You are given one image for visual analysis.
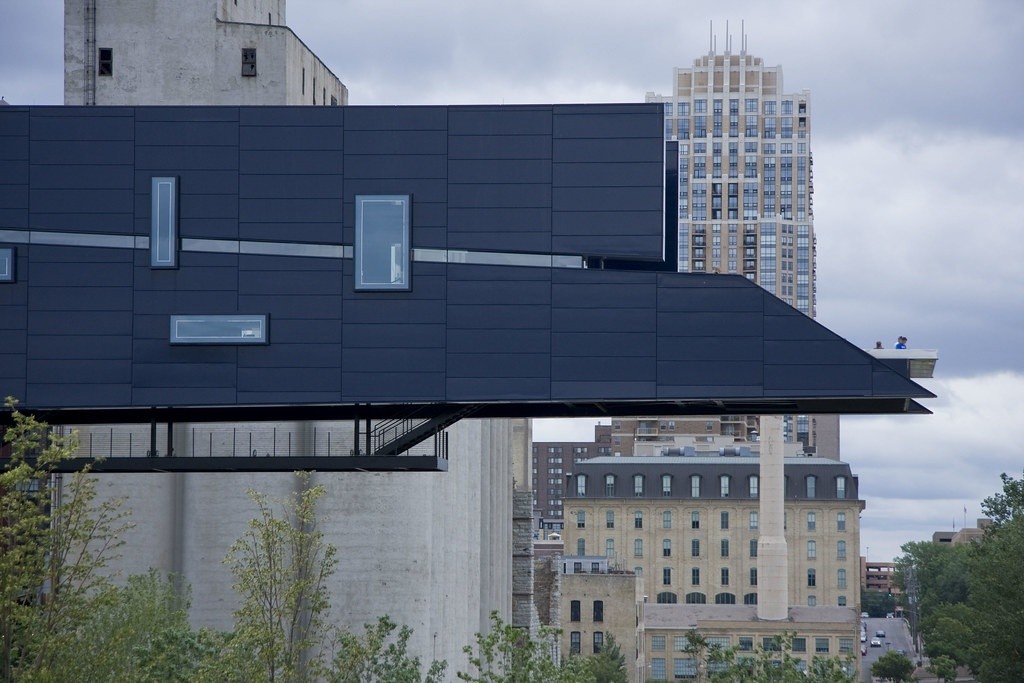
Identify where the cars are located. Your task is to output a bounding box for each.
[876,630,885,637]
[861,645,867,656]
[870,637,882,647]
[860,611,869,618]
[887,613,894,619]
[861,620,867,642]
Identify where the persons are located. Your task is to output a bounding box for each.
[895,335,908,349]
[874,341,883,349]
